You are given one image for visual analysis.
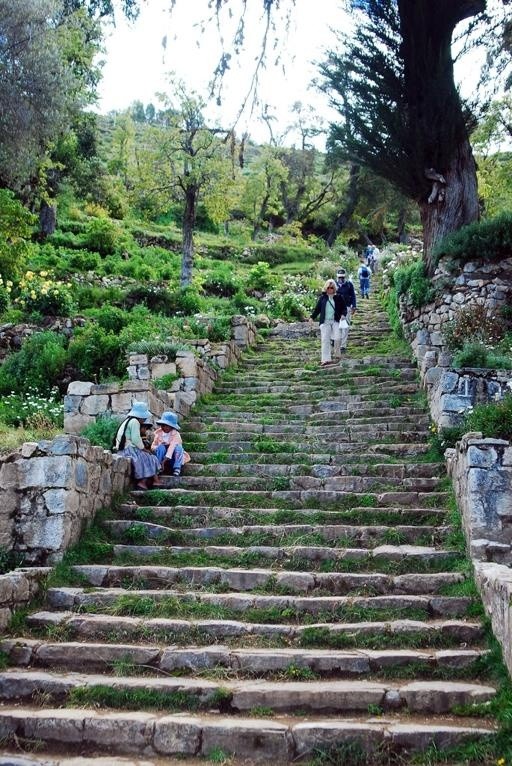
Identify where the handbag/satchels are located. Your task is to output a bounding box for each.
[339,319,349,329]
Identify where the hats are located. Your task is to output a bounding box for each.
[337,269,345,278]
[142,412,154,426]
[126,402,153,418]
[156,412,180,432]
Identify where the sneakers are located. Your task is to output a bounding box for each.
[173,469,180,475]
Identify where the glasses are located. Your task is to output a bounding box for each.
[327,287,333,289]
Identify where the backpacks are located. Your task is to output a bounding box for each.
[362,266,368,277]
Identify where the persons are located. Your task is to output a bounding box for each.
[140,412,154,449]
[116,402,166,489]
[330,269,356,350]
[358,243,380,300]
[309,279,347,366]
[151,412,191,476]
